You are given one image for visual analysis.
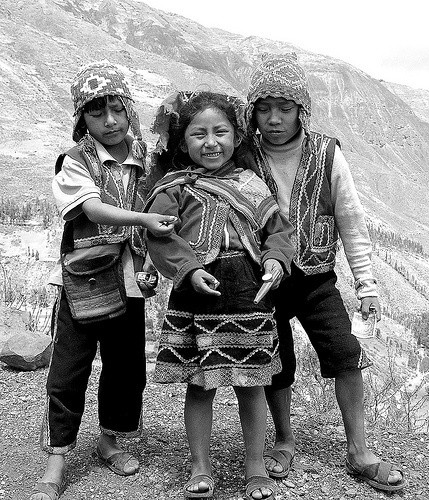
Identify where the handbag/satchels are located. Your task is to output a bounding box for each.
[62,252,127,324]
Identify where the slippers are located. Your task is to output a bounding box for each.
[27,481,67,500]
[262,447,296,477]
[344,458,407,490]
[96,444,139,476]
[243,467,277,500]
[183,469,215,498]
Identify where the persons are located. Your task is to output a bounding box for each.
[28,59,177,500]
[146,88,290,500]
[239,53,407,491]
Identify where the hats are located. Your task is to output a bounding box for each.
[70,62,148,160]
[245,51,312,154]
